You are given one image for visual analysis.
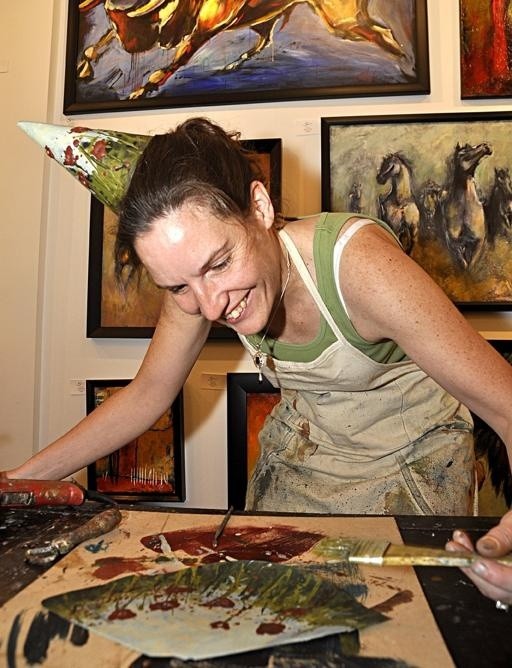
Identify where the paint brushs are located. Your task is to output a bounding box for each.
[214,505,234,539]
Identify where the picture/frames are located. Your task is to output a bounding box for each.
[227,372,281,511]
[86,138,282,339]
[320,110,512,312]
[63,0,430,116]
[85,379,186,502]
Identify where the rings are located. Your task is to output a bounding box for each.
[494,599,509,614]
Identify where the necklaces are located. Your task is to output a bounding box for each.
[244,253,292,380]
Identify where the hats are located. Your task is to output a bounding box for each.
[18,121,154,219]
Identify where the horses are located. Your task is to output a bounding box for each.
[416,178,442,241]
[484,167,511,237]
[439,142,493,276]
[375,152,421,259]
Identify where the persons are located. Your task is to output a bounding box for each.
[0,116,512,616]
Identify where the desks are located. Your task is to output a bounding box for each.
[0,501,512,668]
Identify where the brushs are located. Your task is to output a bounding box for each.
[314,537,512,567]
